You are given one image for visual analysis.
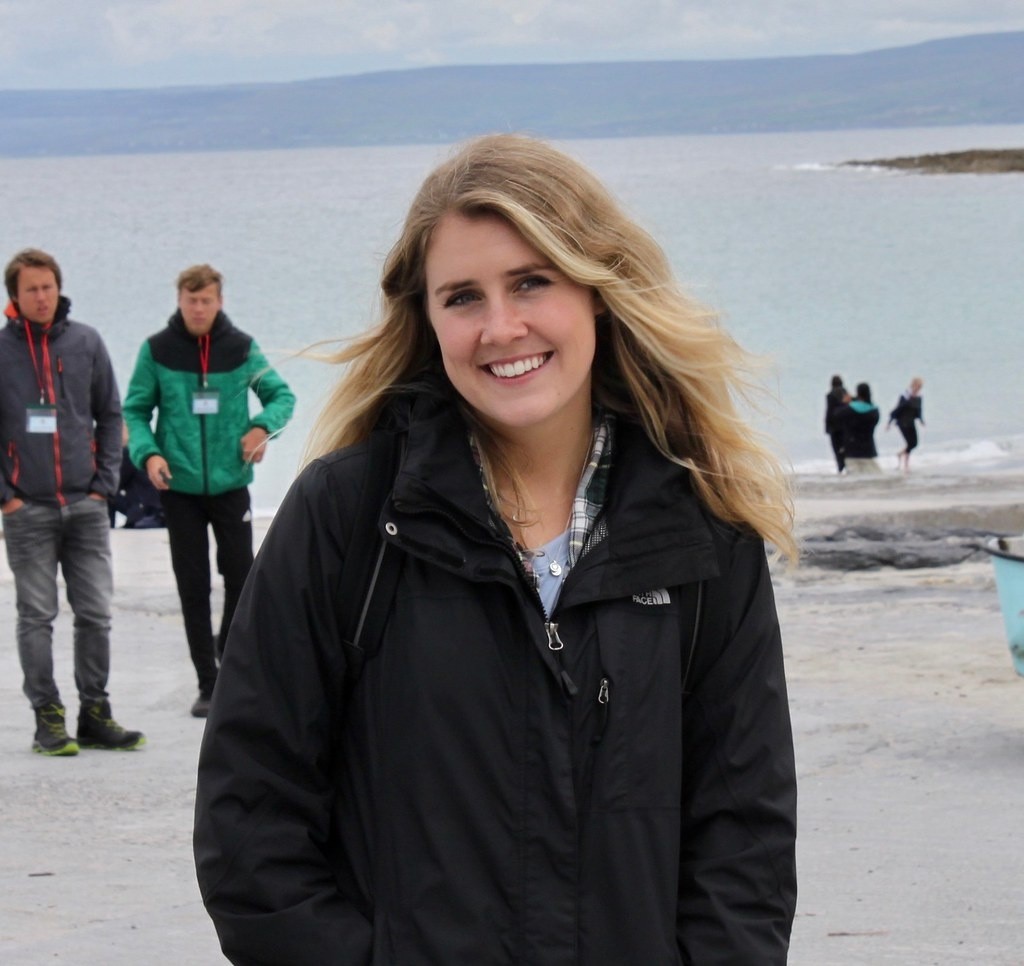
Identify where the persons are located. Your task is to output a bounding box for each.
[124,262,297,717]
[886,378,927,471]
[195,141,798,966]
[0,246,148,757]
[106,406,164,529]
[825,375,851,472]
[831,382,882,474]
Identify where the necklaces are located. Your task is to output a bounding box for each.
[480,420,591,575]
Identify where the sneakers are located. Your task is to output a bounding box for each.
[190,692,212,717]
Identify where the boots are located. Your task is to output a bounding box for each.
[30,704,80,758]
[75,700,146,749]
